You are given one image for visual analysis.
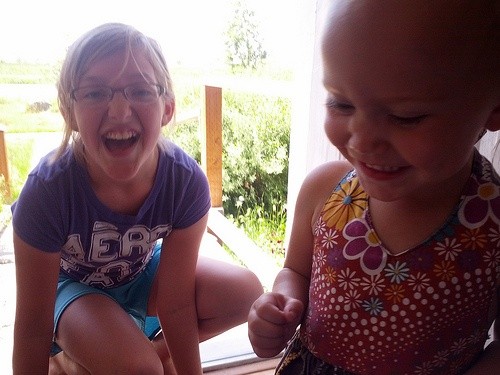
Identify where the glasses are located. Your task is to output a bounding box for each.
[67,82,165,108]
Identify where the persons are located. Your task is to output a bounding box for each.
[10,22,264,375]
[246,0,498,375]
